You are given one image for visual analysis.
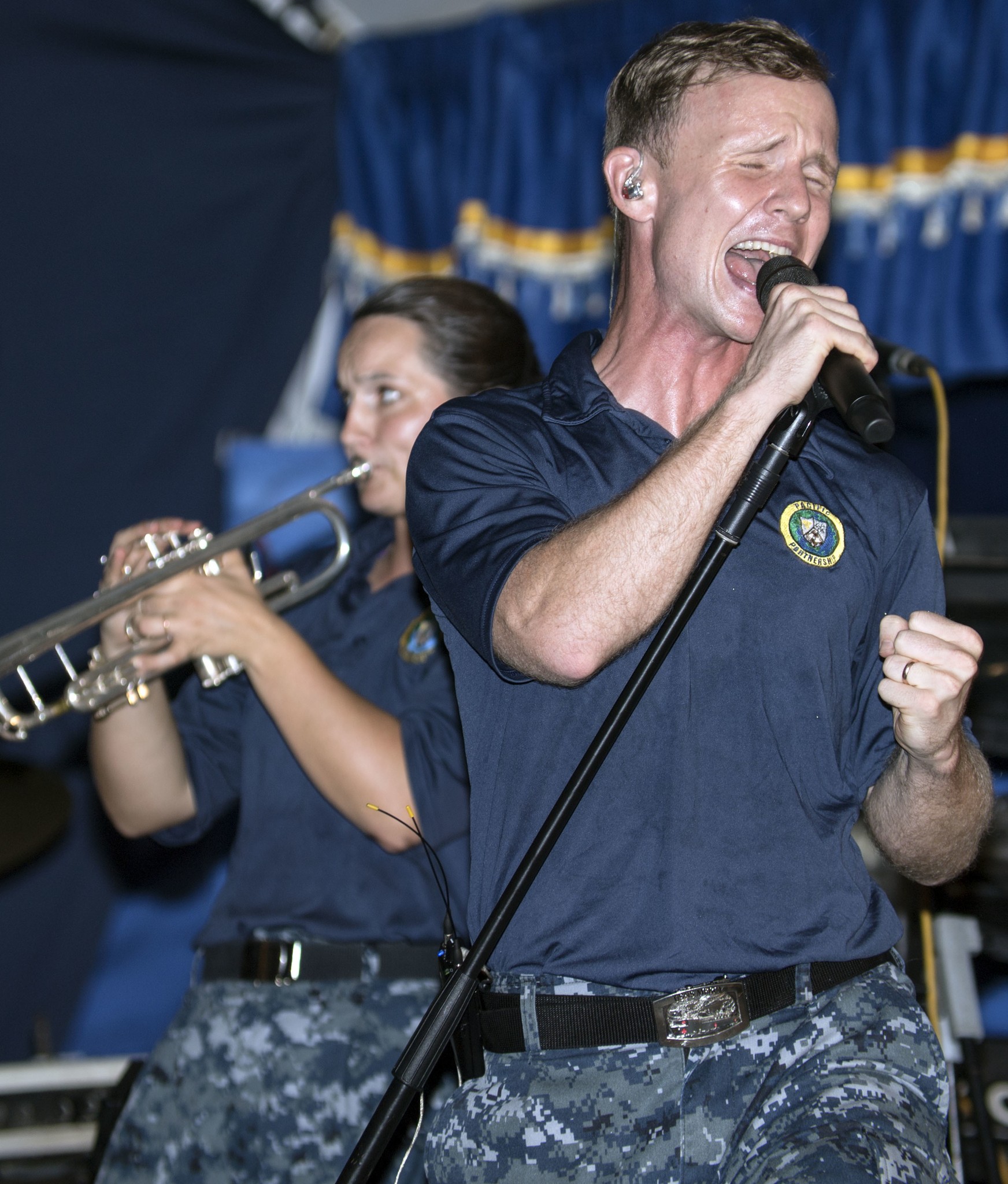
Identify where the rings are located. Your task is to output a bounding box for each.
[161,616,167,638]
[902,659,917,686]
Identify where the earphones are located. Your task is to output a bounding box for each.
[622,177,645,201]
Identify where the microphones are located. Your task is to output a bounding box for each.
[756,255,895,446]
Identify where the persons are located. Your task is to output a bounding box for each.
[407,15,997,1184]
[88,277,546,1184]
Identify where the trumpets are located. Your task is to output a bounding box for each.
[0,454,373,742]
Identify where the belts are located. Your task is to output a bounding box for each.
[203,936,438,984]
[475,947,891,1055]
